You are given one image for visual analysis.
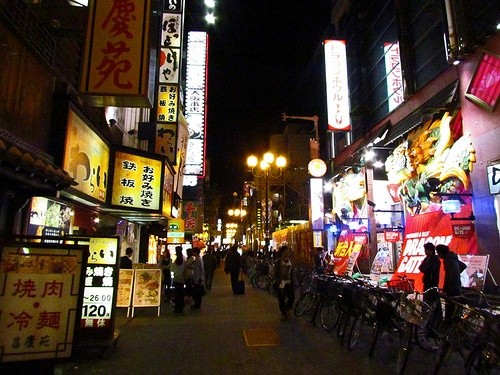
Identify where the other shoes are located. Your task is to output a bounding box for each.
[280,312,288,320]
[191,305,201,309]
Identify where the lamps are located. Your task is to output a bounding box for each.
[247,150,286,178]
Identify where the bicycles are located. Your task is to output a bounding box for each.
[249,251,500,375]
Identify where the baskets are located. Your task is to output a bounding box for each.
[400,299,432,329]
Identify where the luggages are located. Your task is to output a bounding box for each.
[237,271,245,295]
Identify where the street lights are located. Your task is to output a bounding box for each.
[247,149,288,251]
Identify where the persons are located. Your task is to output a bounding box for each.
[118,246,133,269]
[418,243,468,321]
[160,242,335,324]
